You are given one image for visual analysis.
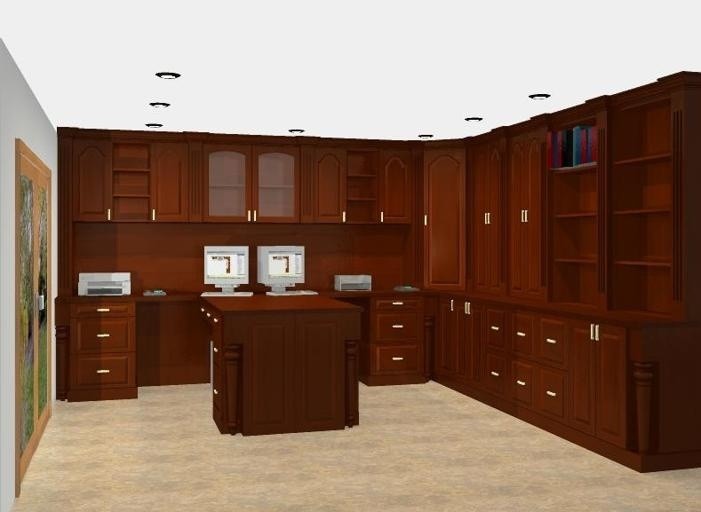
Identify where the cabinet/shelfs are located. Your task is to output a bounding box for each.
[199,295,365,437]
[430,68,698,474]
[58,116,434,400]
